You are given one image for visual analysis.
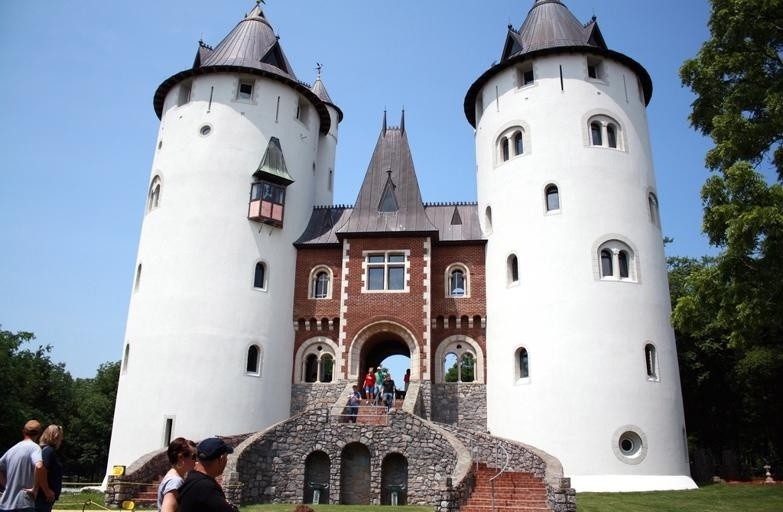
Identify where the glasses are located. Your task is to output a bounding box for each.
[191,452,197,461]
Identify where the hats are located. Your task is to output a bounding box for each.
[197,437,232,460]
[377,366,381,369]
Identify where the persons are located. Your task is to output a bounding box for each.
[348,366,410,424]
[176,438,238,512]
[35,424,63,512]
[158,437,198,512]
[0,419,42,512]
